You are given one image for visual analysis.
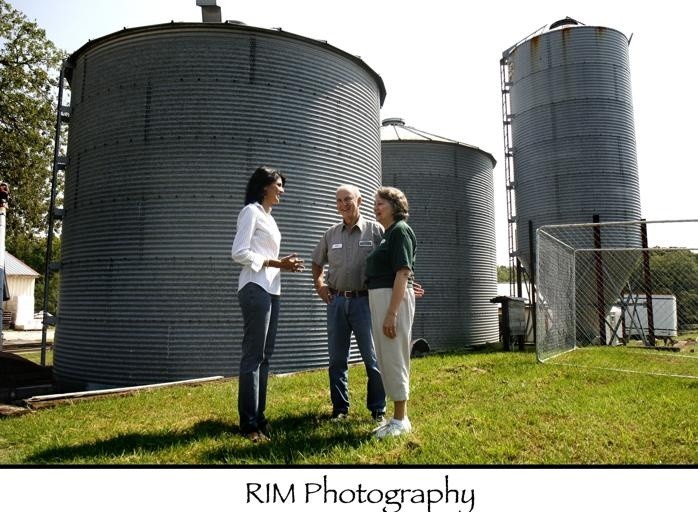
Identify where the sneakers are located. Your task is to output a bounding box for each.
[372,414,387,424]
[373,418,414,440]
[245,428,274,446]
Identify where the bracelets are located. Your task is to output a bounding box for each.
[266,259,270,270]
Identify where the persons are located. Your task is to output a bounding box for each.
[366,186,418,437]
[231,166,306,441]
[310,184,424,423]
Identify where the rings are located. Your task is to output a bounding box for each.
[327,291,331,296]
[389,329,393,333]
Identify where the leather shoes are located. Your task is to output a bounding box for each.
[327,412,349,425]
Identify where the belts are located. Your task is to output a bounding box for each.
[327,286,371,300]
